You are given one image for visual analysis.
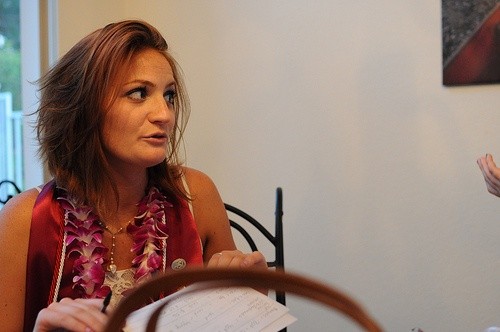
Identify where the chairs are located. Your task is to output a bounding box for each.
[0,180,288,332]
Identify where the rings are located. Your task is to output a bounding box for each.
[216,252,222,256]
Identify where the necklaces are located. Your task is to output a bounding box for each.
[99,220,137,277]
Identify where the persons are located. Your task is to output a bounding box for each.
[477,153,500,198]
[0,20,272,332]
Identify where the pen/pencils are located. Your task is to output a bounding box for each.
[101,290,113,314]
